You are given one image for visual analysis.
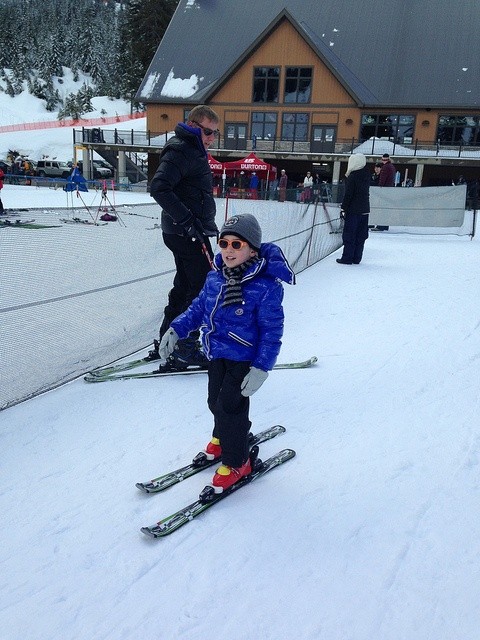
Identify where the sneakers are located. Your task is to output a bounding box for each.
[172,327,208,369]
[213,458,252,490]
[279,200,284,202]
[337,259,344,263]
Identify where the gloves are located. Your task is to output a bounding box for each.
[159,327,179,359]
[240,366,269,398]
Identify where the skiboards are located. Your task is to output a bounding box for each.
[84,330,318,382]
[135,425,296,538]
[59,218,108,226]
[0,219,35,228]
[146,223,161,230]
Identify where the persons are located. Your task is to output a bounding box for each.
[249,172,259,200]
[149,105,219,367]
[378,153,396,187]
[335,153,370,264]
[158,213,296,491]
[279,169,288,202]
[372,163,381,186]
[0,168,6,214]
[372,226,389,231]
[234,171,249,199]
[296,170,331,205]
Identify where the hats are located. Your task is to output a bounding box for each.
[374,163,382,167]
[219,214,261,250]
[382,154,389,161]
[345,153,366,178]
[282,169,285,172]
[322,176,327,180]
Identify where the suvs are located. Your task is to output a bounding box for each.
[77,160,112,179]
[37,161,72,179]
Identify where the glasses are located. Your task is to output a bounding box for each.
[193,120,219,135]
[218,239,248,250]
